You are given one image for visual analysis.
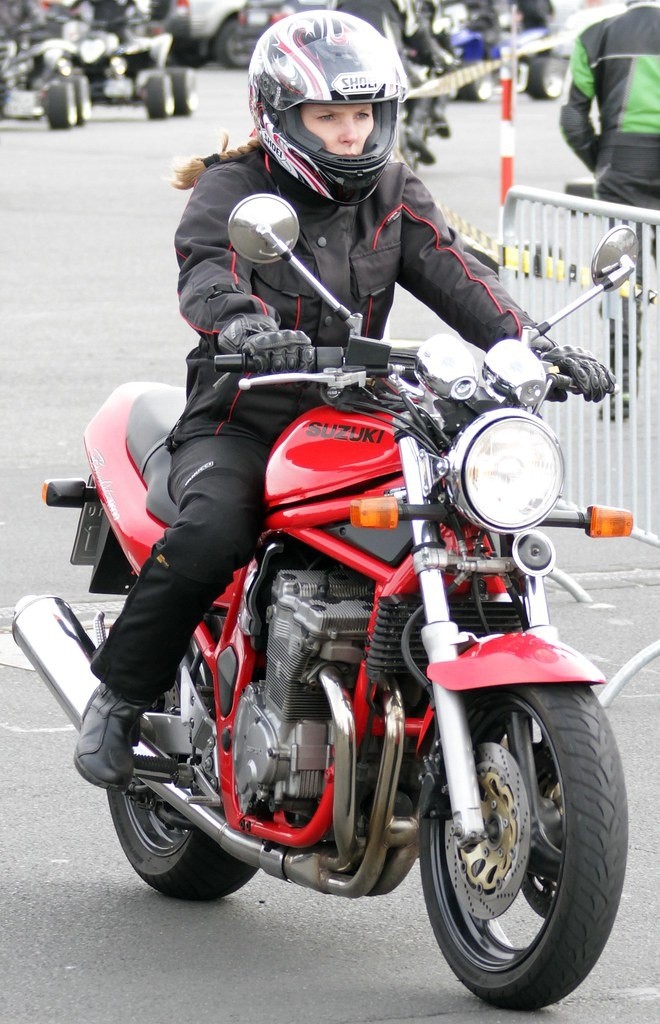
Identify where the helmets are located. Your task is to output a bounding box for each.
[249,10,399,205]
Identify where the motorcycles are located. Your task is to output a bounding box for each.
[12,193,637,1011]
[426,11,565,103]
[43,14,198,131]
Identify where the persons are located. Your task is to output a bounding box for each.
[66,0,136,45]
[0,0,47,92]
[558,0,660,421]
[336,0,456,166]
[75,11,616,784]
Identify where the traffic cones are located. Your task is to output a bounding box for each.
[498,65,514,242]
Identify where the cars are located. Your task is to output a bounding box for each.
[144,1,333,69]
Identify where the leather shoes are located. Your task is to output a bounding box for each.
[74,683,150,790]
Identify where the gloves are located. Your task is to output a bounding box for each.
[216,312,308,389]
[530,331,613,404]
